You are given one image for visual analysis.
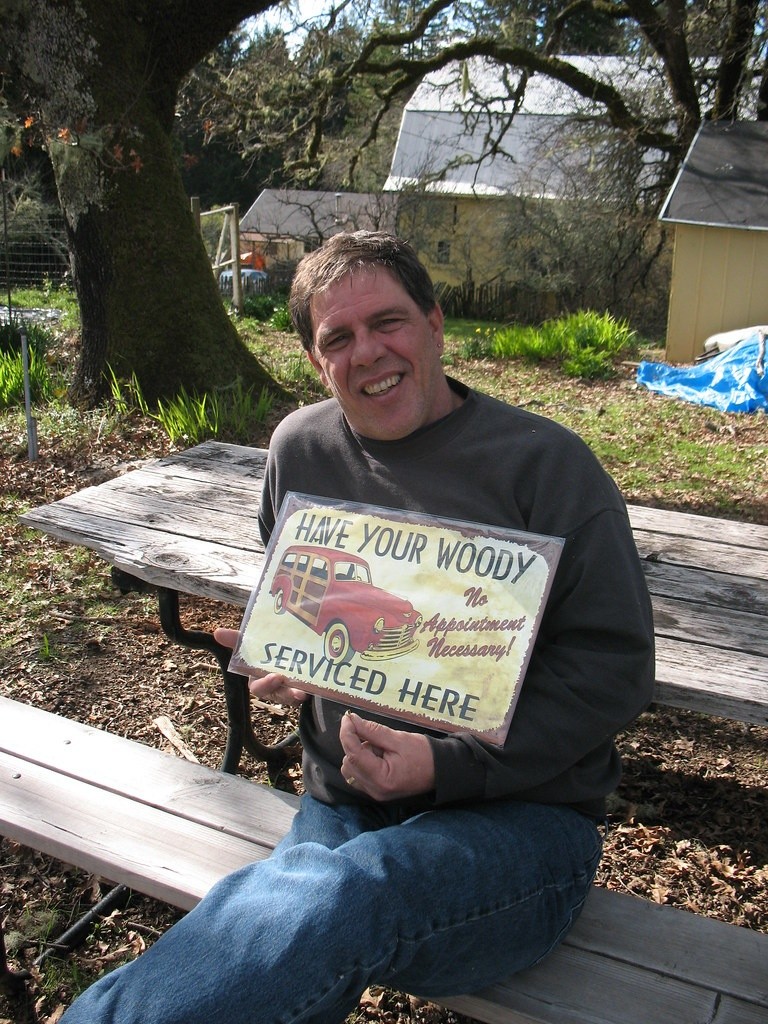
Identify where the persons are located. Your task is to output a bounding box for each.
[52,229,655,1024]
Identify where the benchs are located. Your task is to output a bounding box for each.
[0,696,768,1024]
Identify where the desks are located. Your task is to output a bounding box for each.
[20,441,768,962]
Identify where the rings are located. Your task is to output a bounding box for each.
[346,777,355,784]
